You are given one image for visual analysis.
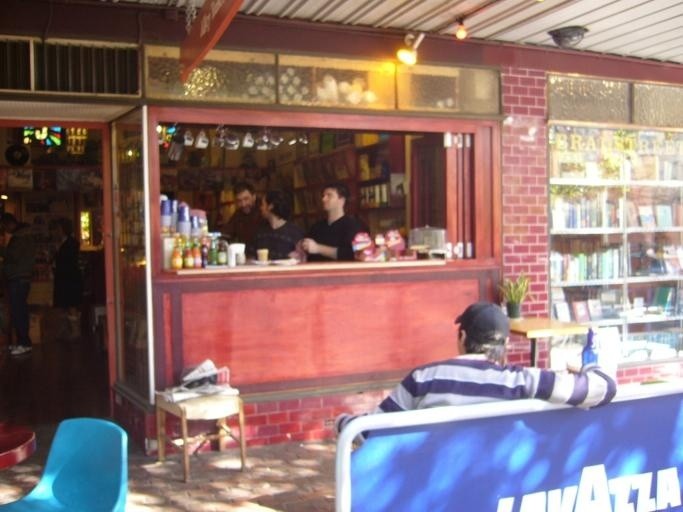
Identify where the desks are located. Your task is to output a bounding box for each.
[0,423,39,476]
[503,315,586,369]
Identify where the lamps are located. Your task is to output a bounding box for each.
[453,16,472,46]
[393,25,428,66]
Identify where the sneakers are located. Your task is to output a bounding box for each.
[9,346,33,355]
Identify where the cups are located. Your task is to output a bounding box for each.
[255,249,268,260]
[159,200,199,237]
[357,183,388,208]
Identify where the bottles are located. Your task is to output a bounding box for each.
[580,329,598,367]
[170,235,235,270]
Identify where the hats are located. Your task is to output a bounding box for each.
[455,302,509,346]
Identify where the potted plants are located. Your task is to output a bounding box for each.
[494,271,532,321]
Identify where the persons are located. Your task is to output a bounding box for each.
[0,212,38,356]
[336,302,616,444]
[44,217,86,344]
[220,182,267,245]
[246,189,307,260]
[295,183,370,261]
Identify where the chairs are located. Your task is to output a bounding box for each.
[0,417,128,512]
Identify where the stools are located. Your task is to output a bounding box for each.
[155,389,249,484]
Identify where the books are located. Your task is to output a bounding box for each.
[549,151,682,351]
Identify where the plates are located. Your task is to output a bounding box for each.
[274,259,299,265]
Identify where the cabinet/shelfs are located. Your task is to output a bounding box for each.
[551,120,683,367]
[168,144,404,223]
